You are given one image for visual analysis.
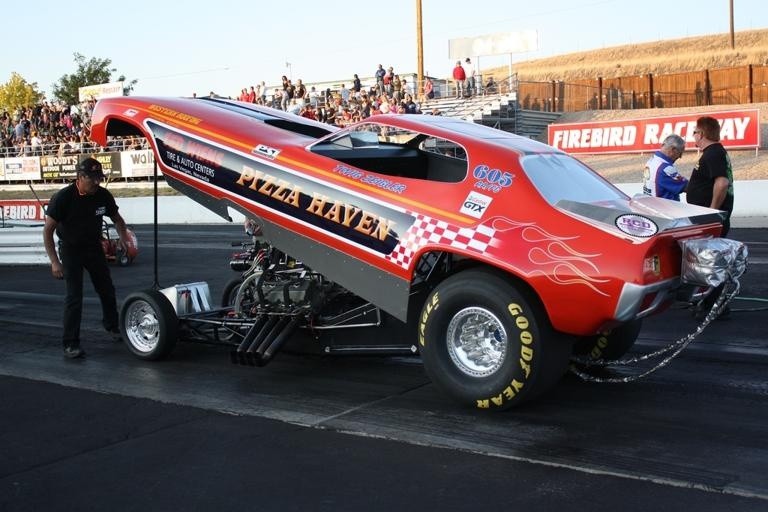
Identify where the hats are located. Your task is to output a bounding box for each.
[78,158,106,178]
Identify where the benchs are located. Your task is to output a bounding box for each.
[411,68,516,131]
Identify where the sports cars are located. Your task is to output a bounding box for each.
[89,95,749,413]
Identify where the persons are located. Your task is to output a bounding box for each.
[0,95,146,183]
[686,117,735,322]
[43,157,129,359]
[642,134,690,311]
[486,76,498,95]
[235,64,442,135]
[453,60,466,100]
[463,59,477,98]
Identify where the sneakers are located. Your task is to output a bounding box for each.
[106,326,124,341]
[63,342,85,358]
[695,307,733,321]
